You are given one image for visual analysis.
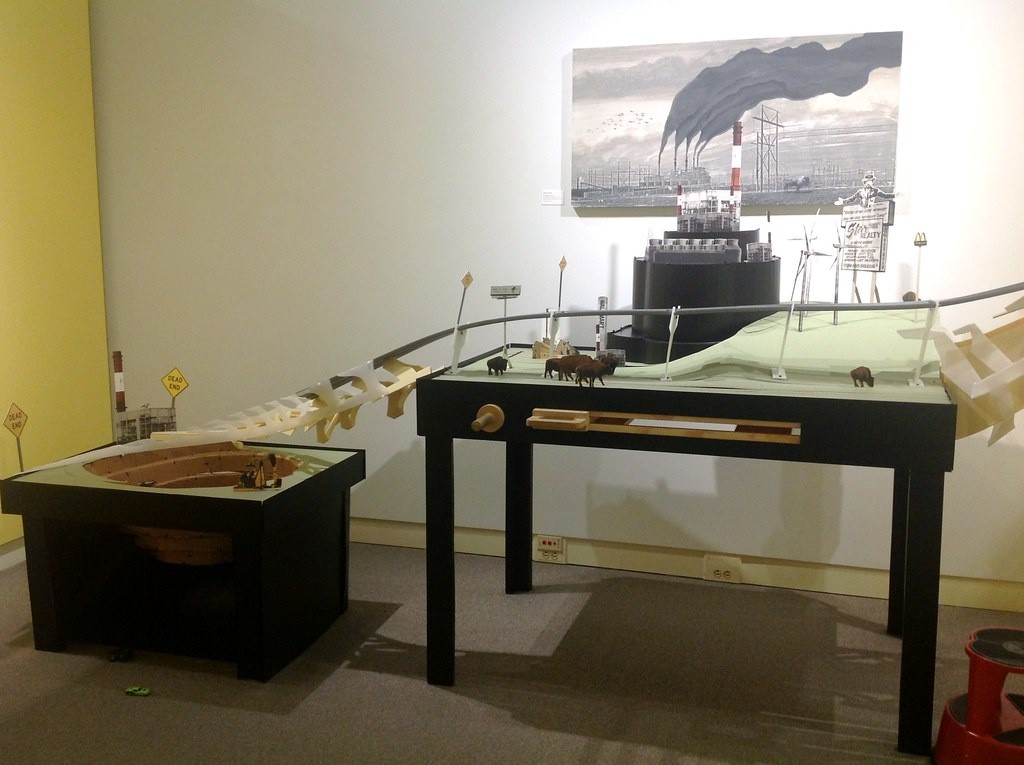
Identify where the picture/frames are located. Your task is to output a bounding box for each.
[572,30,903,208]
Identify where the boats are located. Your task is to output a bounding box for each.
[634,120,781,344]
[784,186,813,193]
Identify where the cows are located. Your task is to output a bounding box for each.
[544,355,617,388]
[487,356,508,376]
[851,366,875,387]
